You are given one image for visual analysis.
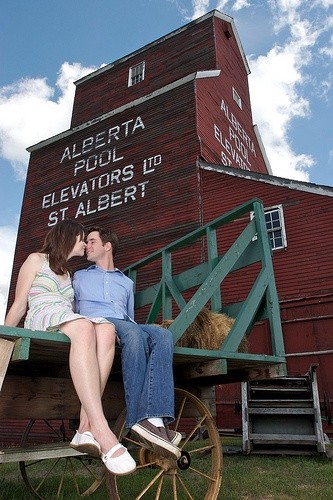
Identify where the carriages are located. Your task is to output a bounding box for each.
[0,197,287,500]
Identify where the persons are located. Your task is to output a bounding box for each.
[71,226,183,461]
[3,217,137,476]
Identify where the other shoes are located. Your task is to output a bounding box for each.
[130,419,181,458]
[70,431,102,456]
[100,441,137,476]
[167,429,181,446]
